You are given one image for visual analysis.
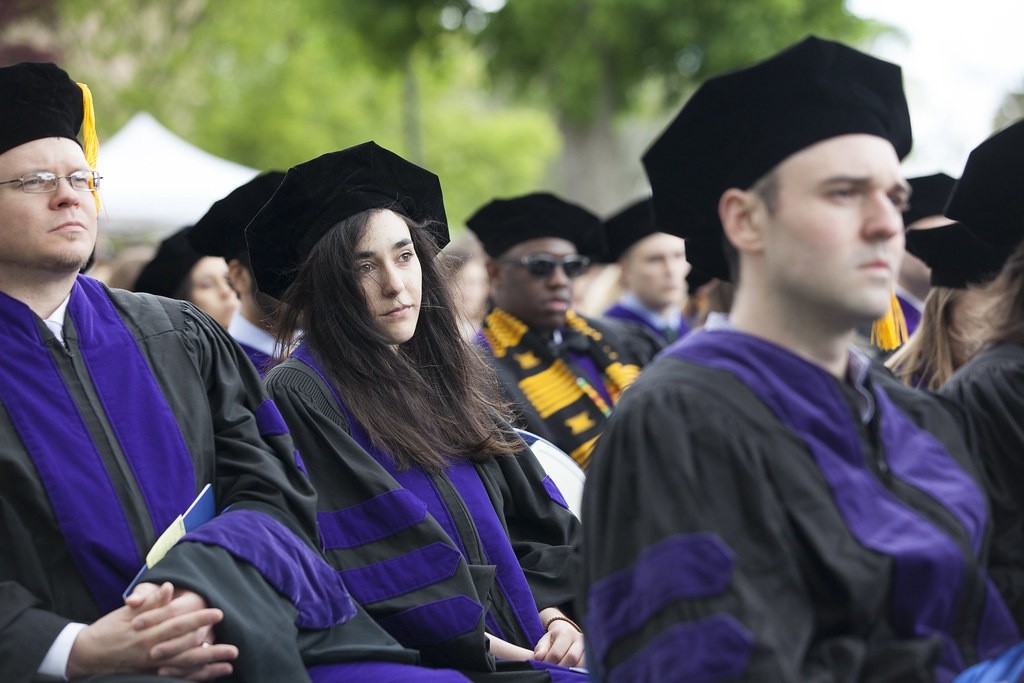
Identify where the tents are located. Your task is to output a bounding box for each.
[85,109,262,290]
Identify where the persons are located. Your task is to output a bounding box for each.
[578,39,1023,683]
[598,191,697,346]
[934,117,1020,626]
[883,216,991,397]
[868,173,967,370]
[440,242,489,346]
[468,186,640,473]
[683,240,740,327]
[123,226,240,337]
[251,137,598,683]
[182,166,313,378]
[0,61,361,683]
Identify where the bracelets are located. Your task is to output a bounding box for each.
[544,618,585,636]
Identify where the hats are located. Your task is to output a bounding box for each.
[1,62,100,213]
[131,140,452,304]
[467,36,1024,289]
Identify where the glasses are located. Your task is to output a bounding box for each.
[498,252,592,278]
[0,171,103,193]
